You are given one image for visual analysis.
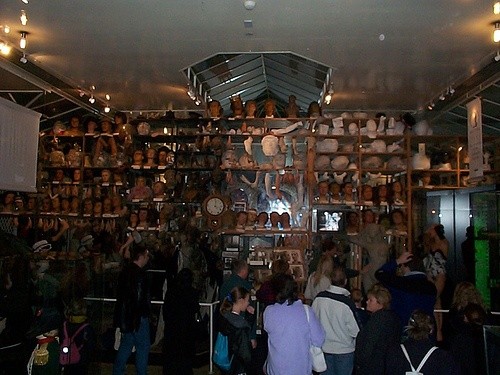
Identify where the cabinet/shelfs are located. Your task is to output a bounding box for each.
[35,112,500,293]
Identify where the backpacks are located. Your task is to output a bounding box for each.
[59,322,89,365]
[212,332,235,370]
[399,344,438,375]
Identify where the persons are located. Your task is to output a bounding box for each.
[1,95,414,279]
[263,276,329,375]
[0,269,51,375]
[162,268,201,375]
[356,284,401,375]
[195,239,454,331]
[113,246,153,375]
[442,281,500,375]
[213,286,261,375]
[311,266,368,375]
[416,224,449,298]
[415,138,500,187]
[28,308,67,375]
[385,308,462,375]
[58,297,96,375]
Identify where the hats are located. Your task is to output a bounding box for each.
[32,240,52,254]
[81,235,93,245]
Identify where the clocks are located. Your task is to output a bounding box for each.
[204,194,227,218]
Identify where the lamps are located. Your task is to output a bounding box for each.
[20,53,27,65]
[494,51,500,62]
[20,10,28,25]
[19,31,29,49]
[324,84,335,105]
[494,21,500,43]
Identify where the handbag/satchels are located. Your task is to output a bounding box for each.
[311,346,327,372]
